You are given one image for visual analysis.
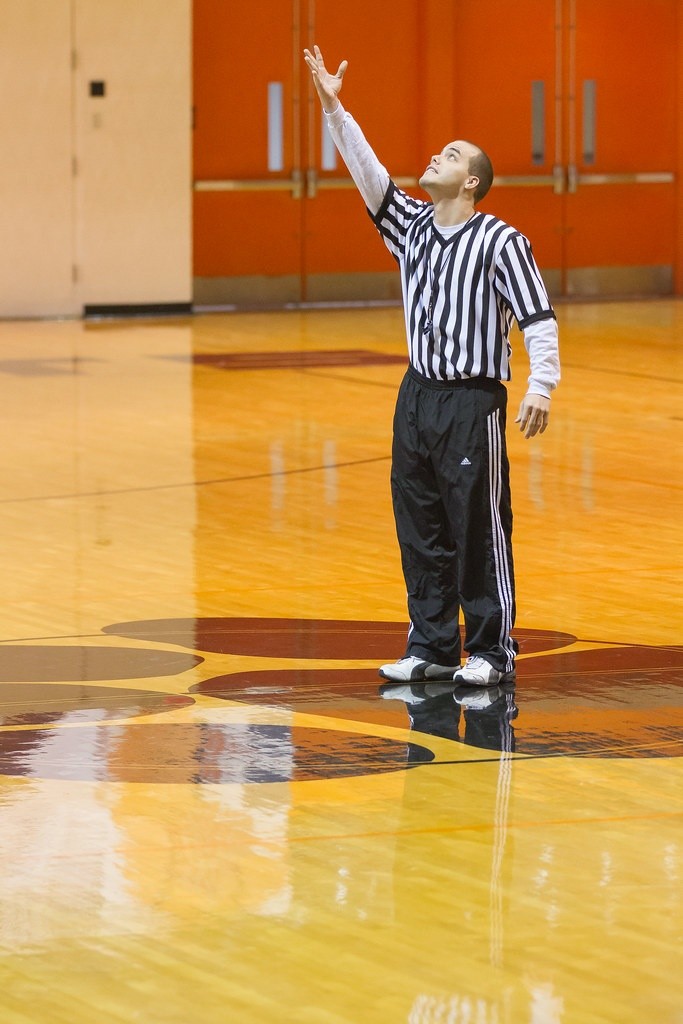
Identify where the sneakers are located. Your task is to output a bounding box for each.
[379,680,460,706]
[379,655,464,681]
[453,655,517,687]
[453,681,516,709]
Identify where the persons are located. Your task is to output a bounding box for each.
[299,44,562,690]
